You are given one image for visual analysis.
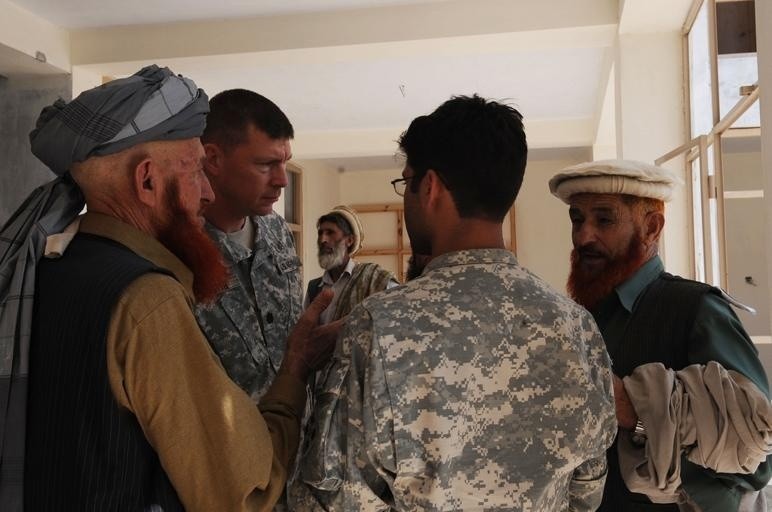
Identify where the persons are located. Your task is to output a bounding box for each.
[305,205,406,323]
[282,93,620,510]
[1,62,351,512]
[549,159,771,511]
[181,92,312,404]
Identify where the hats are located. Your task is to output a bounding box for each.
[330,205,365,255]
[549,159,678,202]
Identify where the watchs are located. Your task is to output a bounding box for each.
[629,416,648,446]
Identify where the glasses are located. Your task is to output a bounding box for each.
[392,169,453,197]
